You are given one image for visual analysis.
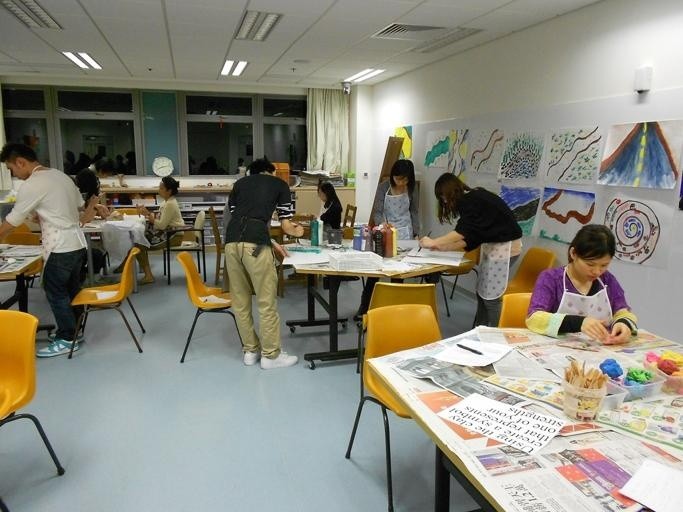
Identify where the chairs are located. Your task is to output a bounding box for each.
[344,302,443,512]
[0,206,206,360]
[419,245,479,316]
[208,206,227,285]
[342,204,357,227]
[496,291,530,329]
[498,248,555,301]
[176,251,246,363]
[1,308,67,510]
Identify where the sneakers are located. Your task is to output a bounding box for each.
[36,334,84,357]
[244,350,260,366]
[260,350,297,369]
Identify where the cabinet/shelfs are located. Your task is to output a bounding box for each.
[101,183,355,245]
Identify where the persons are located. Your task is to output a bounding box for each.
[0,143,90,358]
[355,159,422,324]
[222,200,234,245]
[221,159,304,367]
[74,169,111,281]
[419,171,525,328]
[133,177,185,286]
[524,223,639,345]
[313,181,344,224]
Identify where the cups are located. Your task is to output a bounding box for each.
[105,199,111,205]
[562,374,607,420]
[238,166,246,176]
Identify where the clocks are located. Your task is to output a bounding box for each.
[152,157,174,178]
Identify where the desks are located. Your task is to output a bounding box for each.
[278,235,474,369]
[269,215,322,298]
[366,328,682,512]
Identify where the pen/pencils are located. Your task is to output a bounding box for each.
[456,344,483,355]
[418,230,433,252]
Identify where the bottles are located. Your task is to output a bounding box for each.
[113,194,118,205]
[360,229,370,251]
[316,216,323,246]
[309,216,318,247]
[390,225,397,256]
[346,167,355,187]
[382,223,394,258]
[371,227,385,256]
